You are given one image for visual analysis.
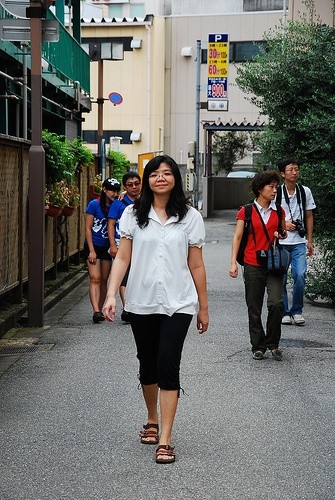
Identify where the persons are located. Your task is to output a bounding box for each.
[273,159,316,323]
[106,171,141,324]
[99,155,209,463]
[84,178,121,321]
[230,170,287,358]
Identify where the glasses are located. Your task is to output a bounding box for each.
[285,167,300,174]
[125,180,141,188]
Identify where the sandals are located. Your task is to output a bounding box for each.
[139,423,159,445]
[156,445,175,464]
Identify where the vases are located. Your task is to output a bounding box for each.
[47,204,60,217]
[63,204,77,217]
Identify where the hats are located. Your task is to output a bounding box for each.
[102,177,121,191]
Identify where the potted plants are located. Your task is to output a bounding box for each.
[89,181,102,199]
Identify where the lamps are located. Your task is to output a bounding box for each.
[130,39,142,49]
[180,46,192,58]
[129,132,143,142]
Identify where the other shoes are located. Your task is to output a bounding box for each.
[292,314,305,324]
[121,309,130,323]
[92,311,105,322]
[271,349,282,360]
[253,351,264,360]
[282,316,292,324]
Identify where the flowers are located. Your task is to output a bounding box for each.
[67,187,81,207]
[44,182,69,206]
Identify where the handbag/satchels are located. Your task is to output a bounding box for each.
[267,244,290,274]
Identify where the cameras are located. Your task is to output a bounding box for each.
[291,219,308,238]
